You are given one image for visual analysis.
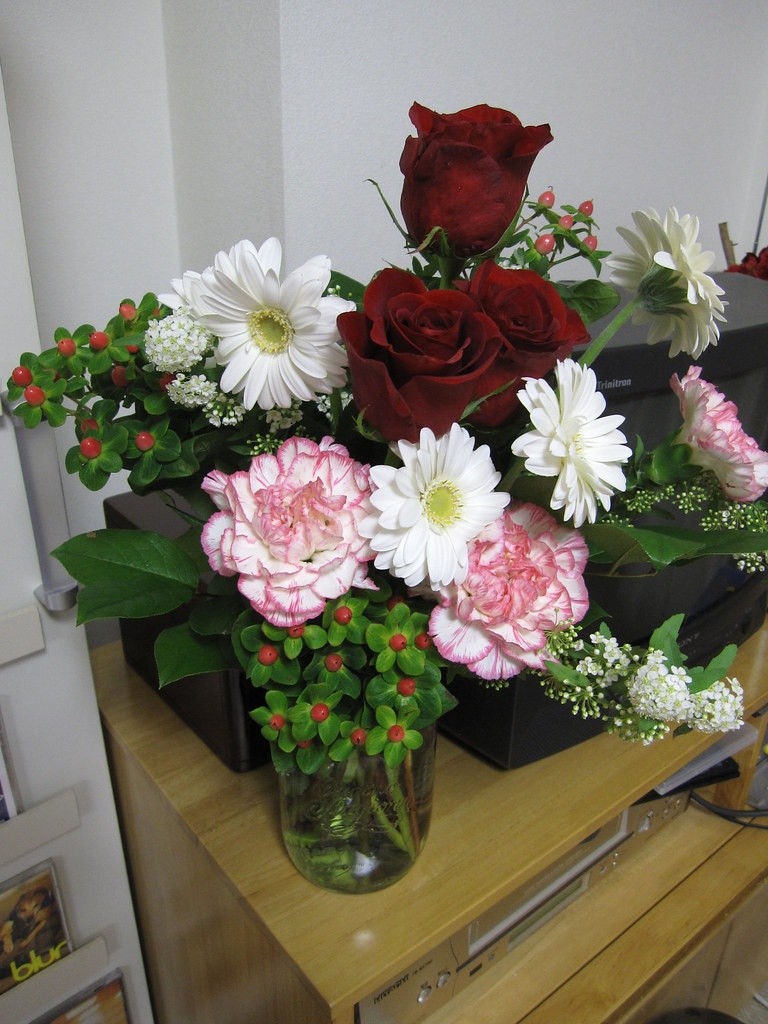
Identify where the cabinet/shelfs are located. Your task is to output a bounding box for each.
[90,616,768,1024]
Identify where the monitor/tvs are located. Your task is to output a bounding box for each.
[343,269,768,770]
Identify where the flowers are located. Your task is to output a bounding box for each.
[0,101,768,892]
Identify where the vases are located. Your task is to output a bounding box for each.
[277,722,438,893]
